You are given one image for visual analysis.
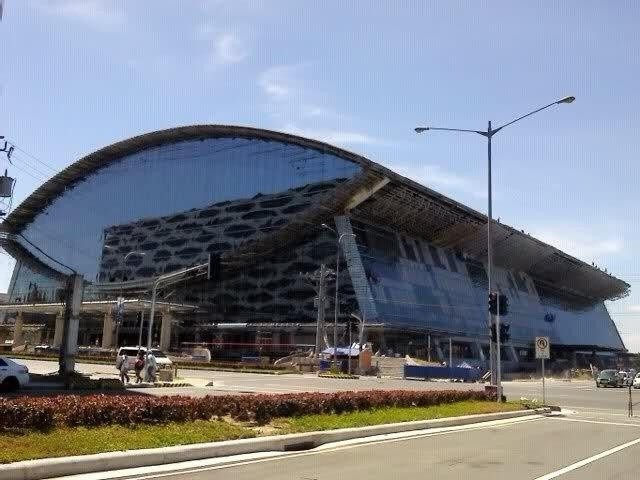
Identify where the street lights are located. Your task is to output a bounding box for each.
[415,93,576,407]
[110,250,147,352]
[322,222,359,372]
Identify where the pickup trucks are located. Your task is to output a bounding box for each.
[35,343,50,349]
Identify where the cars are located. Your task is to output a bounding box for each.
[593,368,640,390]
[0,356,30,395]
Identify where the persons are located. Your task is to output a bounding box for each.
[134,352,143,383]
[119,351,130,383]
[147,350,156,382]
[24,340,28,351]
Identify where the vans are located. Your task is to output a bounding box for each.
[115,346,173,372]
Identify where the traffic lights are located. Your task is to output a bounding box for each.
[489,324,497,344]
[499,294,510,316]
[488,292,497,317]
[499,323,511,345]
